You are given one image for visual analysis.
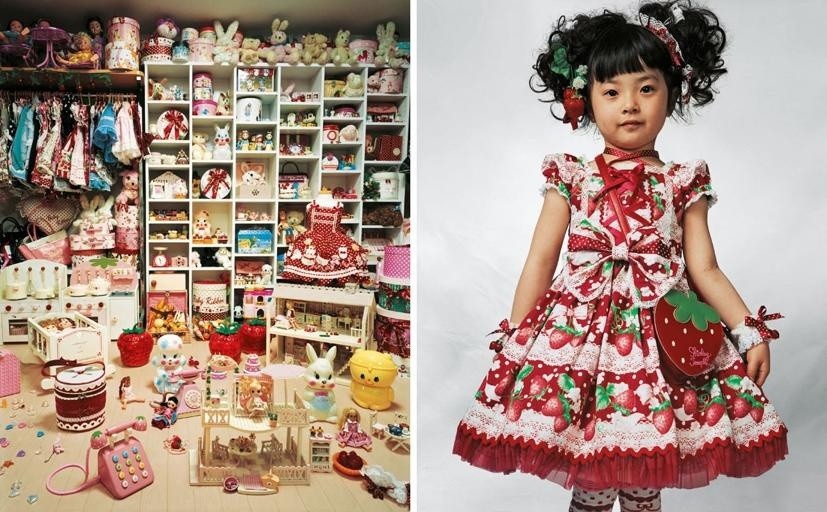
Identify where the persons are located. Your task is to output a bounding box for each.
[452,2,790,511]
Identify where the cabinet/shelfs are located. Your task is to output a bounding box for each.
[1,61,144,84]
[141,58,410,337]
[0,259,141,342]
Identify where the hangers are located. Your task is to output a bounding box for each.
[3,88,136,105]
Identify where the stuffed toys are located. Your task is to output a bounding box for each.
[0,16,106,70]
[349,350,399,413]
[209,318,243,364]
[329,28,358,64]
[301,343,339,424]
[217,90,233,116]
[149,121,231,268]
[118,377,145,409]
[167,85,188,101]
[266,283,377,375]
[375,21,410,68]
[302,30,331,65]
[370,409,410,453]
[148,77,166,100]
[115,171,139,206]
[72,193,118,237]
[0,397,62,502]
[46,416,155,500]
[149,334,206,455]
[308,425,334,474]
[211,19,239,64]
[258,19,301,65]
[144,17,179,48]
[38,319,56,331]
[4,265,135,300]
[117,323,154,368]
[188,353,310,495]
[148,300,224,340]
[240,315,266,356]
[239,37,260,64]
[54,316,76,331]
[26,311,116,391]
[336,407,373,452]
[40,358,108,432]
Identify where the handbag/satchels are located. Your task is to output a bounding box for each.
[0,217,29,268]
[18,217,73,265]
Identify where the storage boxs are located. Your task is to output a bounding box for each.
[156,110,189,139]
[372,304,411,375]
[349,40,378,63]
[201,167,231,198]
[188,37,214,62]
[375,260,412,315]
[68,232,116,250]
[382,245,411,279]
[146,290,191,344]
[105,41,140,71]
[239,69,274,93]
[376,134,402,161]
[237,96,262,120]
[194,71,213,88]
[150,272,185,291]
[237,181,271,199]
[238,229,272,254]
[382,68,404,93]
[193,303,229,321]
[373,171,400,200]
[117,229,138,253]
[192,281,226,303]
[324,124,340,143]
[193,87,212,101]
[113,204,138,230]
[193,100,217,116]
[107,17,140,45]
[141,38,175,62]
[72,252,105,270]
[109,250,139,271]
